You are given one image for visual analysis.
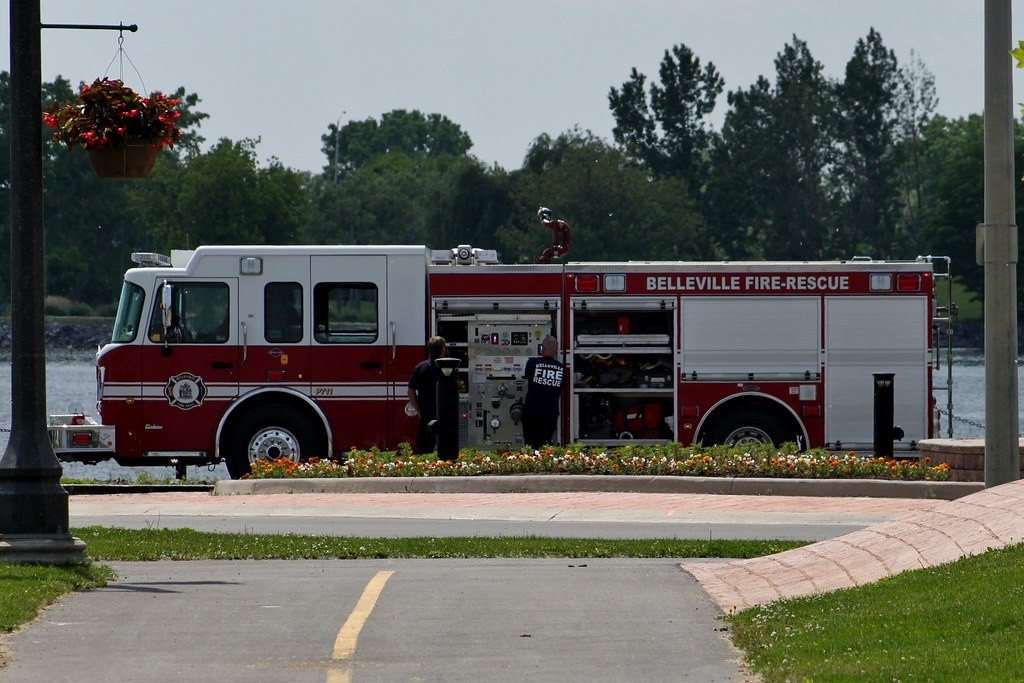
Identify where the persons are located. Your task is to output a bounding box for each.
[196,308,228,341]
[407,336,445,455]
[265,285,299,340]
[521,336,566,457]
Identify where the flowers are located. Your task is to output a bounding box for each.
[43,76,183,152]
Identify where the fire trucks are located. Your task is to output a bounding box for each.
[92,240,959,470]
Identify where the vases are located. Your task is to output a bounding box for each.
[85,145,158,179]
